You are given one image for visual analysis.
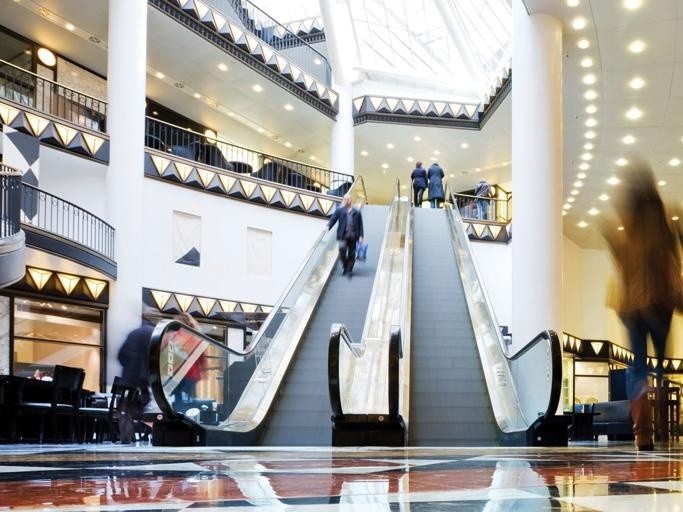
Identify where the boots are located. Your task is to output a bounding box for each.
[630,399,654,451]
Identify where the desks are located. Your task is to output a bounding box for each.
[145,133,164,149]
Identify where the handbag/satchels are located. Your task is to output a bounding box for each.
[354,243,366,262]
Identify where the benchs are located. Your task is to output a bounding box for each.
[575,400,634,440]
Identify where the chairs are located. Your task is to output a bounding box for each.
[12,365,152,444]
[172,141,351,197]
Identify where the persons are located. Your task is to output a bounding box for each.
[600,159,683,451]
[460,199,474,219]
[323,193,364,279]
[426,163,445,208]
[473,178,493,220]
[155,312,211,419]
[409,161,429,208]
[32,369,53,382]
[125,476,187,503]
[114,307,161,445]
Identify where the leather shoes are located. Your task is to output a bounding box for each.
[347,271,352,276]
